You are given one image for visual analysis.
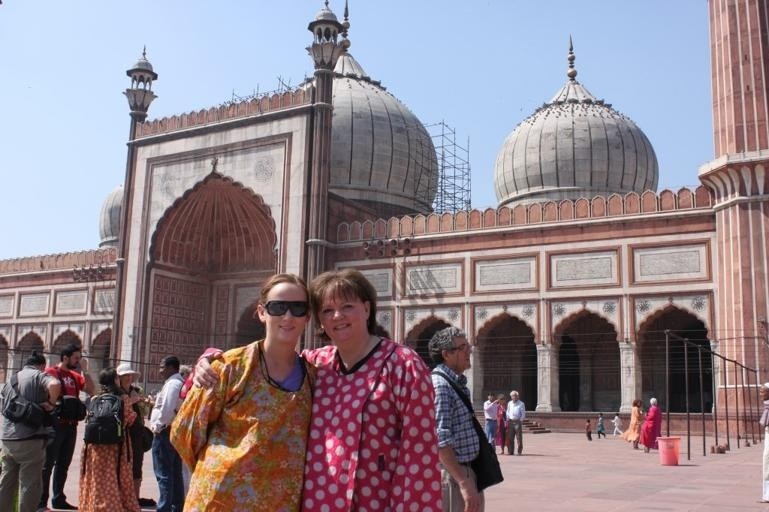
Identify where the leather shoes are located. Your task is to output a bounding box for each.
[52,499,78,510]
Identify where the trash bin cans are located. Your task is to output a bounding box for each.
[655,437,680,466]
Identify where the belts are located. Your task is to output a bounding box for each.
[486,419,497,422]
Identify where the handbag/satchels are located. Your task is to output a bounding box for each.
[469,416,504,494]
[142,425,154,452]
[2,392,47,430]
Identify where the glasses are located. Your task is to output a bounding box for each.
[261,300,311,318]
[452,343,466,352]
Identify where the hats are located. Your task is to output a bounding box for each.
[116,363,141,383]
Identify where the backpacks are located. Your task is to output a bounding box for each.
[83,391,130,488]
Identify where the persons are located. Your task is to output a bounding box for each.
[169,274,320,512]
[0,343,206,512]
[640,398,662,452]
[610,412,623,436]
[483,389,526,455]
[597,413,606,439]
[428,327,485,512]
[756,383,769,504]
[620,399,644,449]
[585,419,592,443]
[180,269,445,512]
